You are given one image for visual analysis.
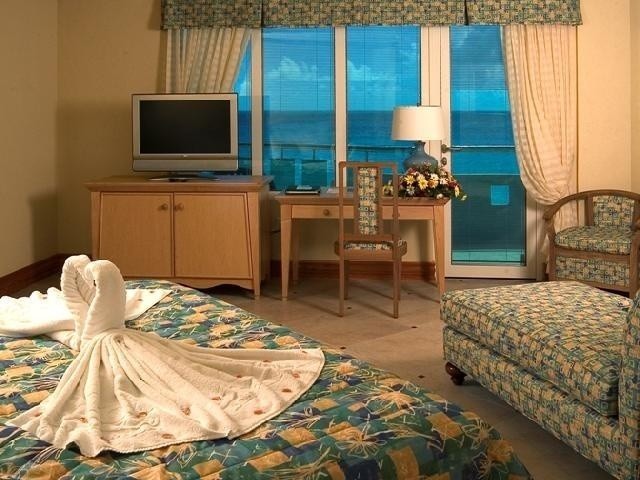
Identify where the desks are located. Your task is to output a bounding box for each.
[272,185,453,308]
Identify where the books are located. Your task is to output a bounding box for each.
[284,184,320,194]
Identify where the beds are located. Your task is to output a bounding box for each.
[1,276,535,479]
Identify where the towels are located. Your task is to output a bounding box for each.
[0,252,177,354]
[2,258,327,459]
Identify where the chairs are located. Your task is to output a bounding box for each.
[334,160,407,319]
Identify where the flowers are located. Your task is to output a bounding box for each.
[382,170,468,203]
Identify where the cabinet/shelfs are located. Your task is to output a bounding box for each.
[83,173,277,300]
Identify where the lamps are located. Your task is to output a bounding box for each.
[389,105,449,173]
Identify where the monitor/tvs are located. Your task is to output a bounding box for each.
[132,93,237,182]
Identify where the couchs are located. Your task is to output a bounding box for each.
[436,274,640,480]
[542,189,639,300]
